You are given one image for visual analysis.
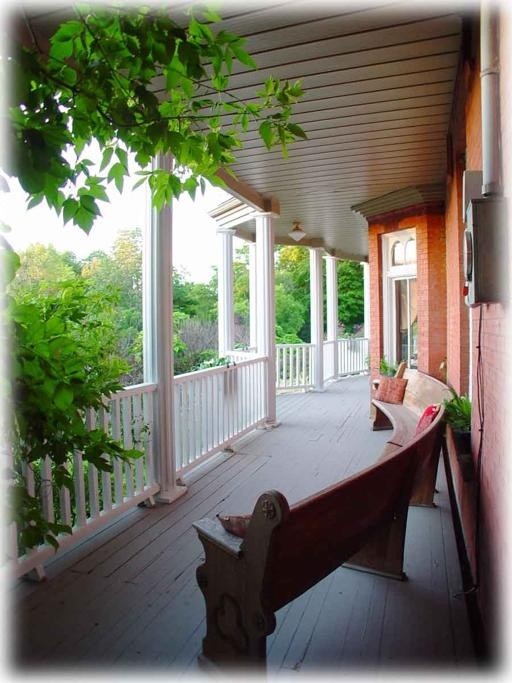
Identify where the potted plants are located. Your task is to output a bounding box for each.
[443,395,474,451]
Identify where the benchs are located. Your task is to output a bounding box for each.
[192,367,454,681]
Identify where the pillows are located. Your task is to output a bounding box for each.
[374,373,408,404]
[415,401,436,437]
[217,509,253,536]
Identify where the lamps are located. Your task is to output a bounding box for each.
[287,221,306,241]
